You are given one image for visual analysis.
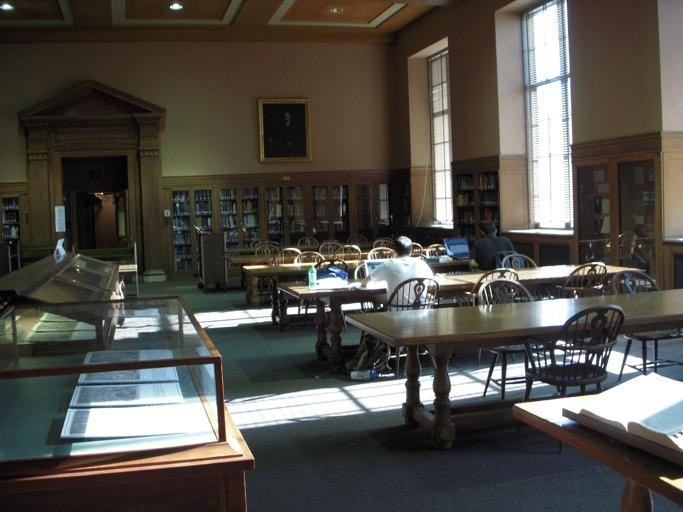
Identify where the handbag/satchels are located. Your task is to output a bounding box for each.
[304,264,348,282]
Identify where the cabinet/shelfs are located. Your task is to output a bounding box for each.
[571,132,683,290]
[216,173,264,248]
[163,175,216,275]
[354,168,400,239]
[264,171,310,244]
[0,254,126,357]
[451,156,529,252]
[1,182,30,260]
[310,170,354,242]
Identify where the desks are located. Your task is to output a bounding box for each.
[447,264,641,306]
[344,288,683,448]
[0,396,255,512]
[514,394,683,512]
[277,277,473,375]
[242,256,476,332]
[230,247,446,266]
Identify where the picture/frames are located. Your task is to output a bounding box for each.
[254,97,313,164]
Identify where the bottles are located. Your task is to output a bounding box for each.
[308,263,318,286]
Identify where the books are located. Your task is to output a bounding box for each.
[640,172,656,209]
[2,197,21,240]
[455,170,497,238]
[592,195,611,235]
[578,372,683,453]
[170,186,349,270]
[426,255,448,262]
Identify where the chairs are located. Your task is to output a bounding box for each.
[297,237,319,251]
[556,264,607,299]
[478,279,555,400]
[347,234,369,246]
[502,254,551,302]
[294,251,325,318]
[319,242,344,260]
[426,244,447,256]
[368,247,393,260]
[354,263,384,343]
[495,250,520,269]
[255,245,279,256]
[304,259,348,331]
[334,245,362,260]
[412,243,423,254]
[612,270,683,381]
[464,269,519,306]
[269,248,301,265]
[361,277,440,374]
[516,305,625,451]
[373,240,394,250]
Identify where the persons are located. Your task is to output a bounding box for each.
[470,219,516,306]
[362,235,434,353]
[268,110,302,151]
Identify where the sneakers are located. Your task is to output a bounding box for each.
[390,346,408,359]
[417,345,429,356]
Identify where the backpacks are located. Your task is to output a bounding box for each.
[346,335,393,374]
